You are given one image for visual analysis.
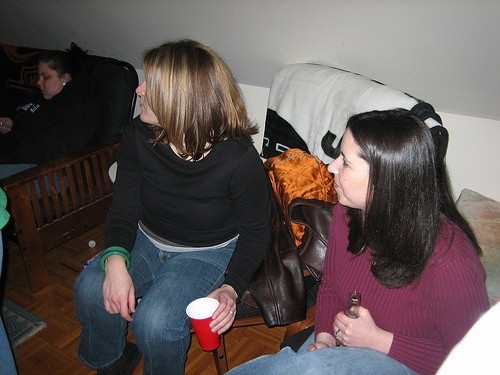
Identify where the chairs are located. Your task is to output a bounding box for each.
[187,63,450,375]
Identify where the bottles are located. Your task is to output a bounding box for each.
[336,292,361,346]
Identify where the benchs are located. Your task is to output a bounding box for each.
[0,44,138,294]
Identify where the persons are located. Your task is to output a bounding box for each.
[223,108,489,375]
[0,188,18,375]
[75,39,271,375]
[0,43,104,210]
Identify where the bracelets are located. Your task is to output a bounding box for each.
[100,247,130,271]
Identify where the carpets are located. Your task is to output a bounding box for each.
[0,296,48,353]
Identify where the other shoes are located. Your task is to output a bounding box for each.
[97,341,142,375]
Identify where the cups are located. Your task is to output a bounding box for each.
[186,297,221,351]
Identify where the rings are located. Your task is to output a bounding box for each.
[335,329,341,340]
[230,310,234,316]
